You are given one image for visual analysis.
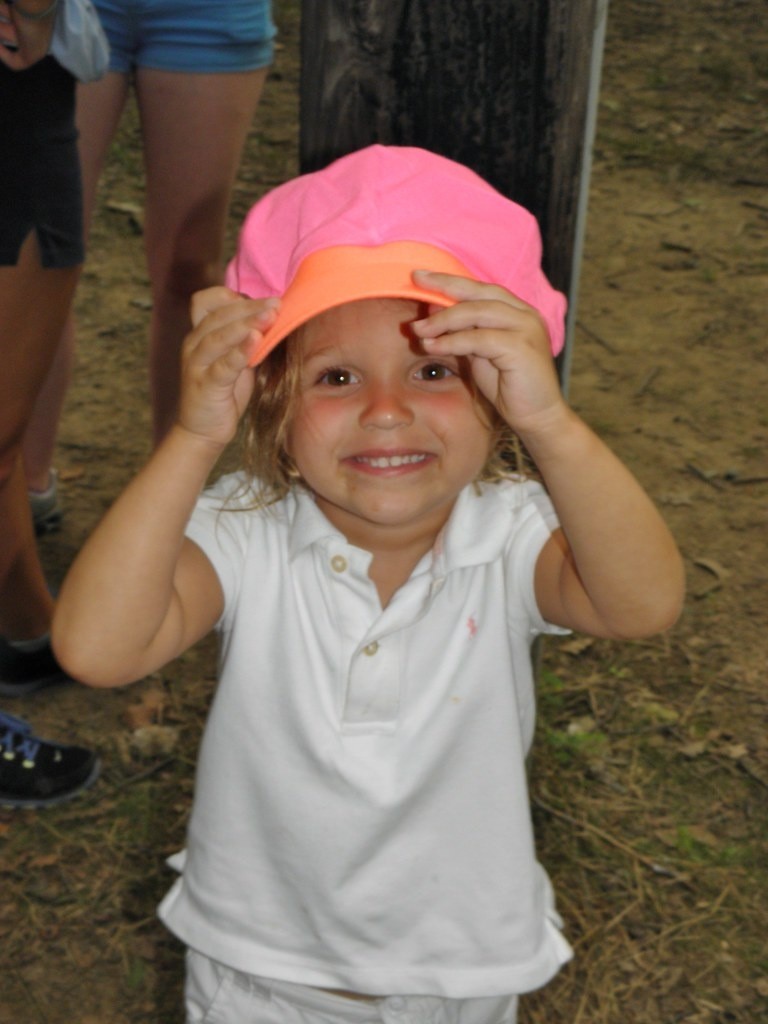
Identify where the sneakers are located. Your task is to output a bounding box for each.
[0,711,104,809]
[0,637,70,694]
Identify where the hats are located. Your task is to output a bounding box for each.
[225,143,566,360]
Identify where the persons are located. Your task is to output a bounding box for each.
[52,143,687,1024]
[1,1,282,811]
